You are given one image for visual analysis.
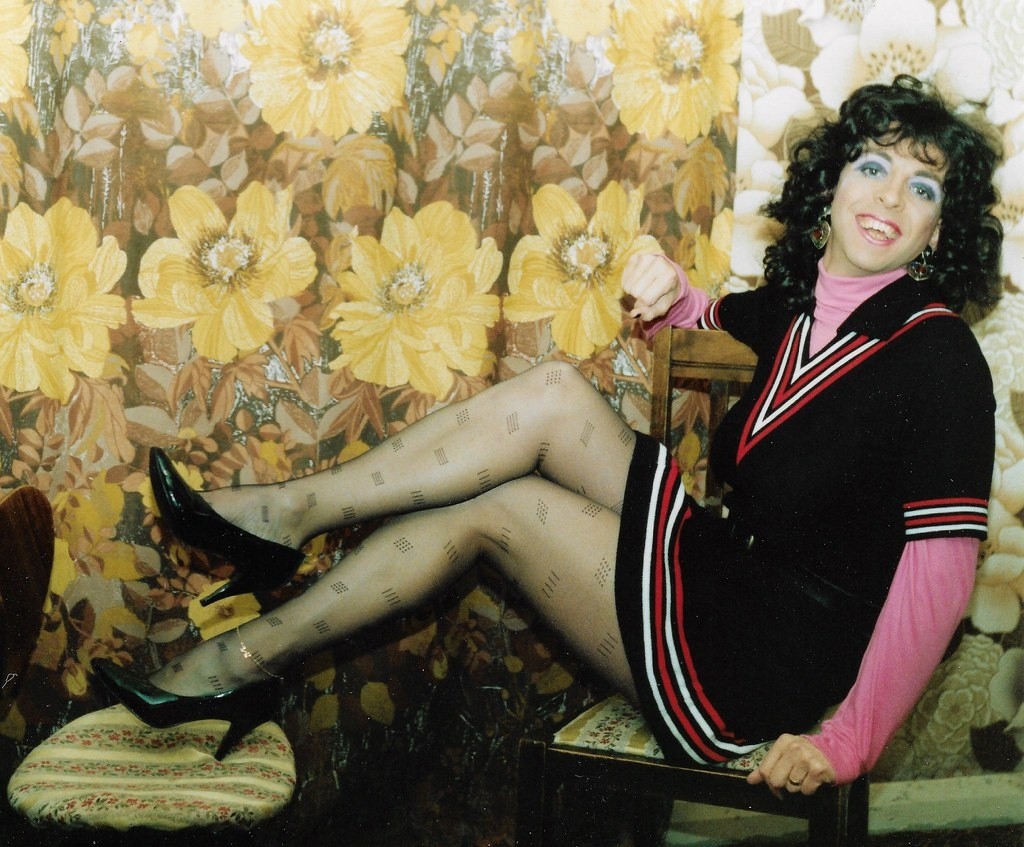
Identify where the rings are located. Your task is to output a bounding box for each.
[789,776,802,786]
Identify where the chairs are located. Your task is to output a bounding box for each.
[0,485,296,847]
[518,329,872,847]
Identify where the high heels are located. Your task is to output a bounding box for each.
[146,447,309,607]
[89,657,285,762]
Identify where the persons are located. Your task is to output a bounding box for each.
[102,75,1002,798]
